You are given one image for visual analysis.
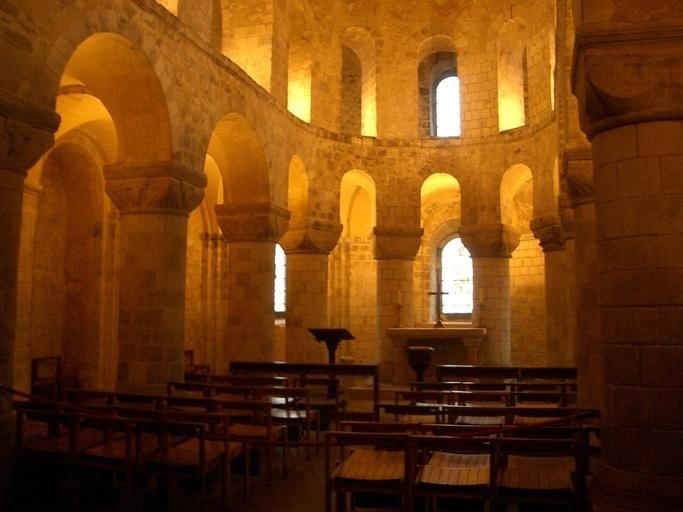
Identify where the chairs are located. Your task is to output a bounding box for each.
[8,361,600,512]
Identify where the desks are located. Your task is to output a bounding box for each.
[387,327,486,385]
